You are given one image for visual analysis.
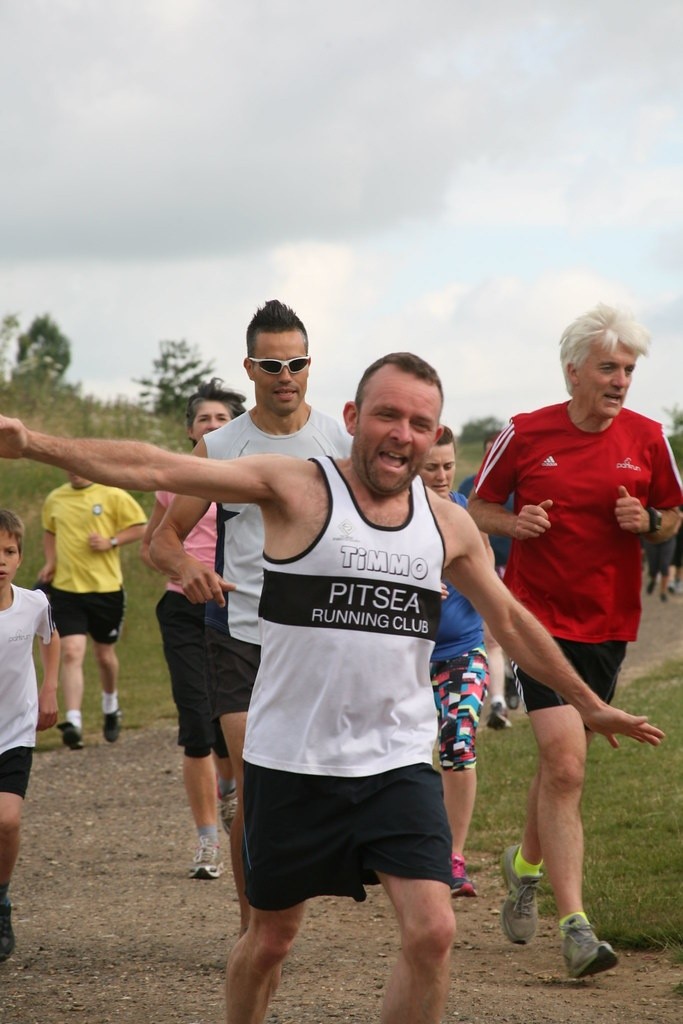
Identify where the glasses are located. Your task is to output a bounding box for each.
[248,356,310,374]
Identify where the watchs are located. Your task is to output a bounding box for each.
[636,507,663,536]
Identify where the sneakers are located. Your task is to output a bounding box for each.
[448,852,478,898]
[499,843,546,945]
[558,914,620,979]
[188,836,228,878]
[219,787,238,834]
[0,899,17,964]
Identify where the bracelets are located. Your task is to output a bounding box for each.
[109,537,118,548]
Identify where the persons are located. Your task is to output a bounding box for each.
[641,505,683,604]
[421,427,518,898]
[520,303,683,979]
[38,470,147,751]
[0,509,61,962]
[0,353,664,1024]
[140,378,246,879]
[149,300,353,941]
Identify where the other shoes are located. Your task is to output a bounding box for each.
[659,592,667,602]
[101,700,123,743]
[56,721,84,750]
[485,701,511,730]
[503,663,520,710]
[668,580,677,594]
[646,580,657,594]
